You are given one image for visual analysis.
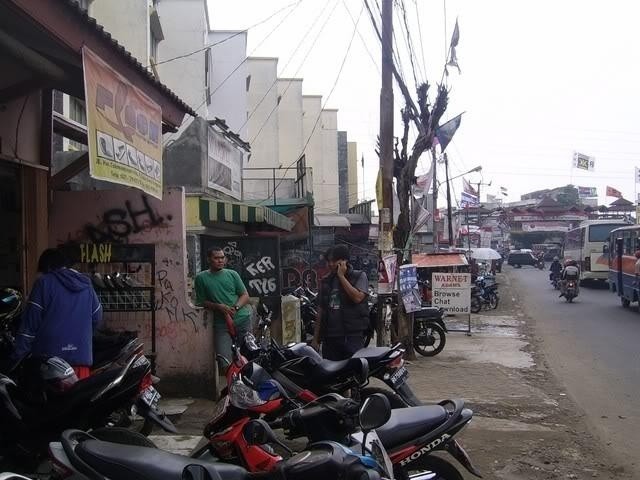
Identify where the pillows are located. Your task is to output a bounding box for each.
[565,260,577,266]
[553,256,559,262]
[15,353,79,402]
[0,287,24,325]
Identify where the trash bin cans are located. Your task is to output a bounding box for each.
[232,305,241,310]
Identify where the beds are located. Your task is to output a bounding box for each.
[470,247,502,272]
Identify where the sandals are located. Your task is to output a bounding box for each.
[602,223,640,309]
[562,218,635,289]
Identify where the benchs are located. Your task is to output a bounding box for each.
[635,168,640,184]
[572,151,596,173]
[606,186,623,199]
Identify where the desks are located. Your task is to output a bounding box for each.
[433,163,483,253]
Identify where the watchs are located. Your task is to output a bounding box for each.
[564,280,579,303]
[537,258,547,271]
[550,271,563,289]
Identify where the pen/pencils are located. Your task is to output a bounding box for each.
[507,252,539,268]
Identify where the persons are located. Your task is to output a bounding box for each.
[311,243,371,399]
[0,239,104,401]
[558,260,580,298]
[558,259,580,280]
[190,246,254,399]
[631,250,640,292]
[548,255,563,285]
